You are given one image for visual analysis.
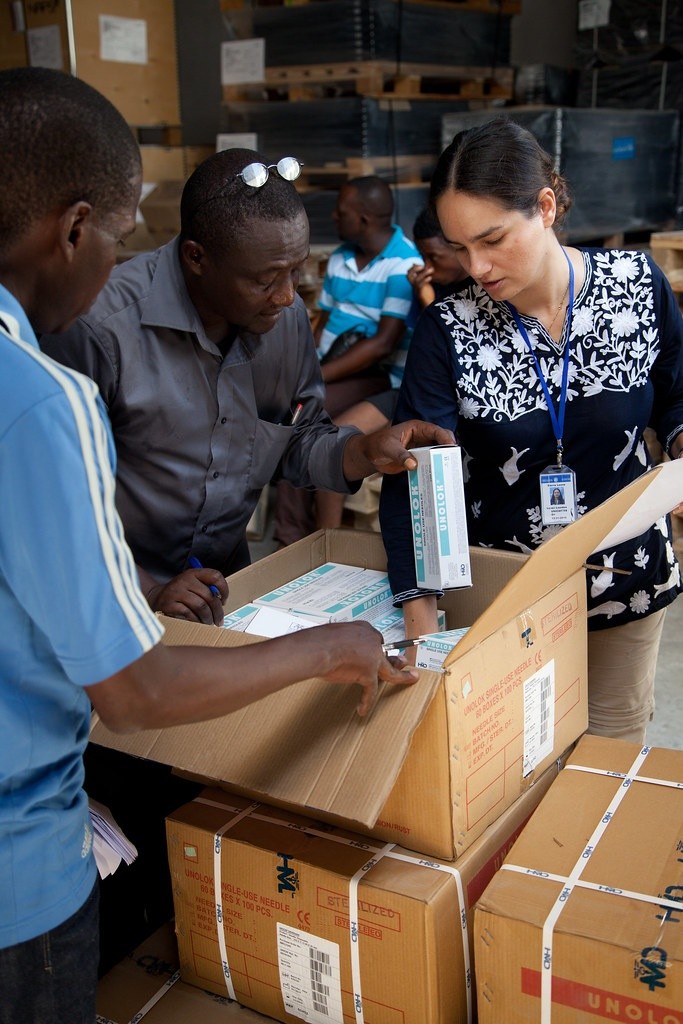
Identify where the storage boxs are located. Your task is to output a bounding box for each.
[0,0,683,1024]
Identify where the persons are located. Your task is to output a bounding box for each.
[40,147,456,626]
[550,488,564,505]
[379,120,683,742]
[275,175,477,553]
[0,65,419,1024]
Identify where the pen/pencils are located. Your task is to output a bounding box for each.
[382,639,426,654]
[291,404,304,427]
[189,555,224,602]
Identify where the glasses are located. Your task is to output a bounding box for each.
[197,156,304,213]
